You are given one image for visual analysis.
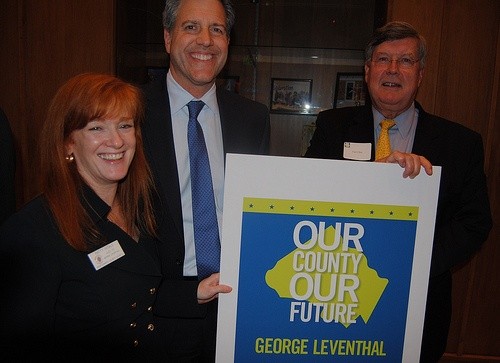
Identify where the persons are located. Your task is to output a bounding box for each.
[0,110,17,219]
[305,18,493,363]
[120,0,273,363]
[0,70,232,363]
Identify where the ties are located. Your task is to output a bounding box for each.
[185,101,221,276]
[374,119,396,160]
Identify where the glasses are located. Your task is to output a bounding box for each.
[371,55,421,67]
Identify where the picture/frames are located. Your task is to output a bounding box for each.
[333,72,367,111]
[268,78,313,116]
